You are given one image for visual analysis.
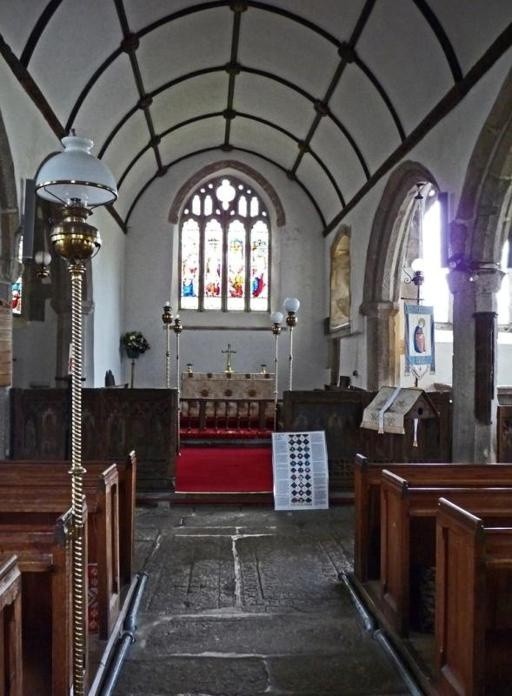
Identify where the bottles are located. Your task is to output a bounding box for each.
[107,370,115,386]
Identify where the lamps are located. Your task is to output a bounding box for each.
[172,314,183,388]
[282,298,300,390]
[34,132,119,664]
[163,301,173,386]
[270,312,282,431]
[410,257,424,302]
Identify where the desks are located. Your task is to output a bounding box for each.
[177,371,274,434]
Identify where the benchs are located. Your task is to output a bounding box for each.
[0,449,137,696]
[351,452,511,695]
